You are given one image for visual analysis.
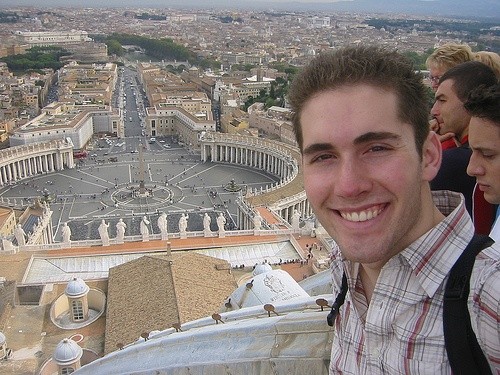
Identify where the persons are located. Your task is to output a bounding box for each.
[115,218,127,235]
[98,219,110,238]
[0,138,64,246]
[62,223,71,242]
[232,242,322,269]
[7,148,249,230]
[209,134,302,230]
[157,211,167,232]
[289,40,500,375]
[425,42,500,242]
[178,212,189,231]
[203,212,211,231]
[140,216,150,235]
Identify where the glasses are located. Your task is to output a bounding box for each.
[428,74,442,83]
